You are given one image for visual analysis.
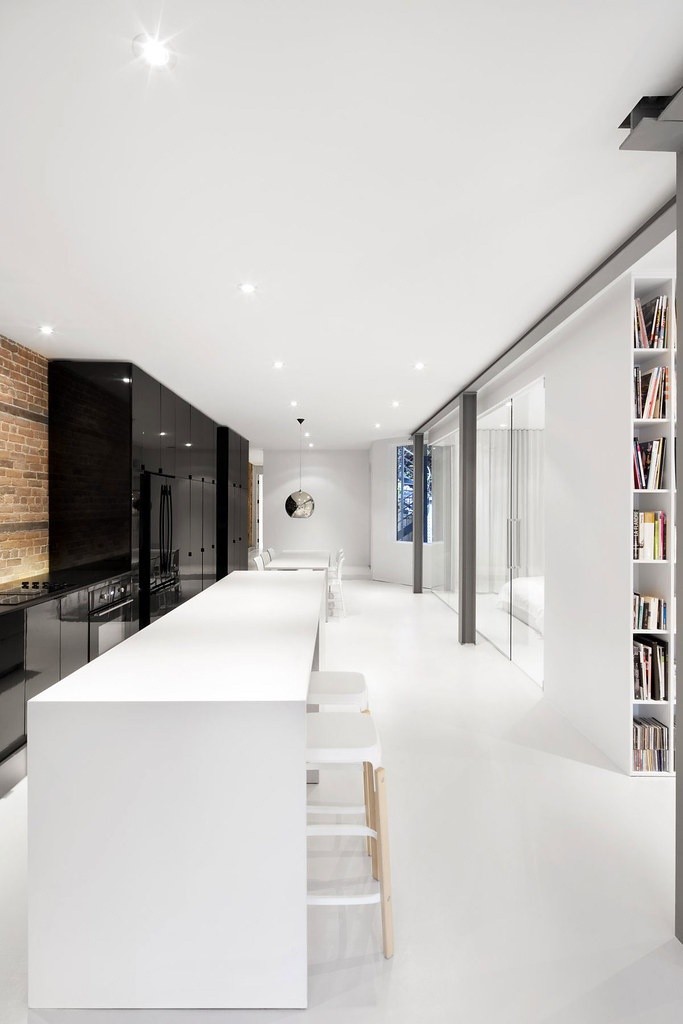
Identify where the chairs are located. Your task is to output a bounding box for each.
[151,558,162,608]
[155,556,176,605]
[170,549,180,603]
[326,548,346,618]
[253,556,264,571]
[267,547,275,561]
[260,551,271,570]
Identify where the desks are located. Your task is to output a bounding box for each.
[131,548,181,593]
[264,549,331,623]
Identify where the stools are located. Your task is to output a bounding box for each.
[306,710,394,961]
[307,670,373,856]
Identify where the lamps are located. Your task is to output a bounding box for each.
[285,419,315,518]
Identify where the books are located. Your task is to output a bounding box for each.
[633,633,669,701]
[634,365,670,420]
[633,716,670,772]
[633,510,667,560]
[634,294,671,349]
[633,591,667,630]
[633,437,667,489]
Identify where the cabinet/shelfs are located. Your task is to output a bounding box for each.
[23,588,89,744]
[216,426,248,581]
[190,479,217,597]
[542,270,677,777]
[176,395,217,484]
[47,361,176,477]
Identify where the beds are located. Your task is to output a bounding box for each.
[497,576,544,635]
